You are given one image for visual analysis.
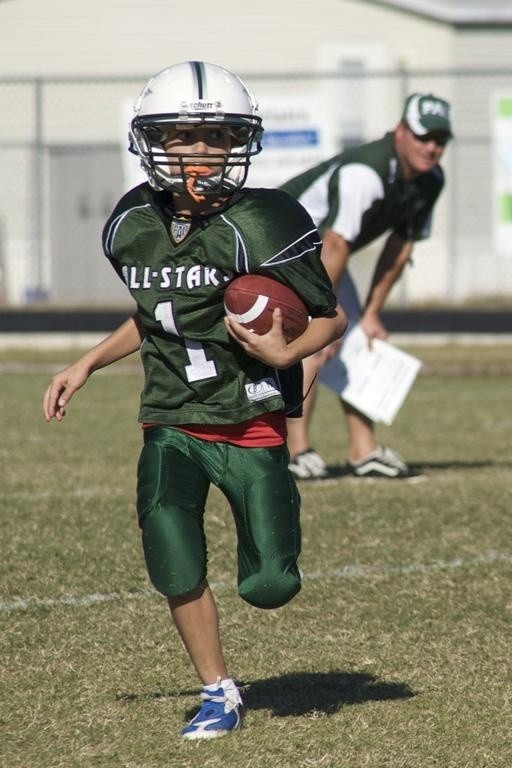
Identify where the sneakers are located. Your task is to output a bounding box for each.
[349,441,413,479]
[183,678,244,743]
[285,450,327,477]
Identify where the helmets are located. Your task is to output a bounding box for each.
[126,61,260,222]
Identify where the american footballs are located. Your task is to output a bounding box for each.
[224,276,308,344]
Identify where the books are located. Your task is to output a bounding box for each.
[318,319,425,427]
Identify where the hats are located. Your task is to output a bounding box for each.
[403,94,454,141]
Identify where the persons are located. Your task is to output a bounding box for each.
[275,93,458,480]
[40,58,348,744]
